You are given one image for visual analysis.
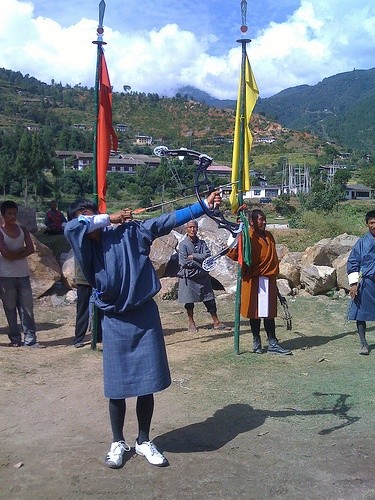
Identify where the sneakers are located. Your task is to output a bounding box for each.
[134,437,170,467]
[103,440,131,469]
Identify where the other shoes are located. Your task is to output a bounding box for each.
[24,333,36,346]
[358,344,372,355]
[74,340,84,348]
[213,322,231,330]
[13,343,18,347]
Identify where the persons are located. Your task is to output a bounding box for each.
[0,200,46,349]
[74,253,102,348]
[224,208,292,355]
[177,219,231,333]
[44,201,70,236]
[346,210,375,354]
[64,190,222,469]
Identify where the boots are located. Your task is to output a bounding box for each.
[250,318,264,354]
[264,317,292,355]
